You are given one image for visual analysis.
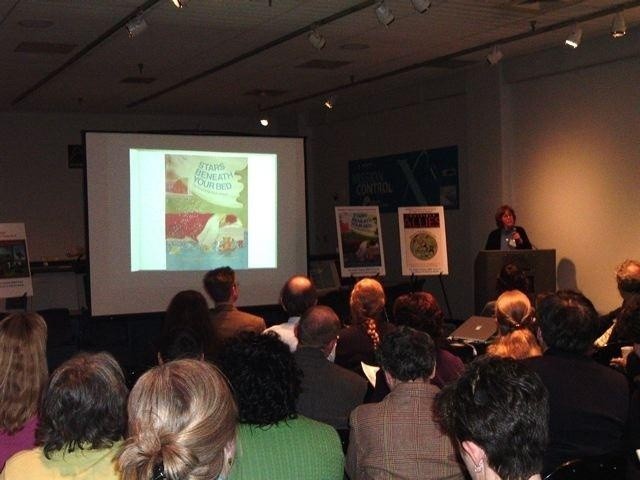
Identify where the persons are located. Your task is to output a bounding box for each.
[485,205,532,250]
[2,350,132,479]
[112,351,242,480]
[2,309,51,472]
[150,255,640,480]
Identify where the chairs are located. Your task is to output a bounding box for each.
[542,456,630,478]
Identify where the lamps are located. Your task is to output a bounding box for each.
[374,3,397,31]
[306,24,329,52]
[485,44,508,65]
[564,20,583,50]
[606,11,629,40]
[172,0,189,12]
[318,93,338,114]
[410,0,433,15]
[124,6,149,40]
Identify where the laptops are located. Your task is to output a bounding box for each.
[447,316,498,346]
